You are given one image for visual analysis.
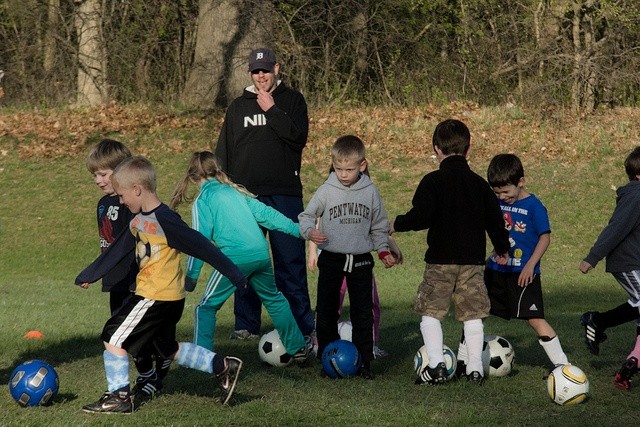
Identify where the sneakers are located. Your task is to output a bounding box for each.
[580,311,607,356]
[131,377,164,410]
[156,342,179,381]
[414,362,448,385]
[82,390,135,414]
[230,329,259,342]
[209,356,243,405]
[357,360,375,380]
[614,357,637,390]
[456,365,486,386]
[293,336,315,363]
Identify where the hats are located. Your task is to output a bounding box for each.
[247,48,276,73]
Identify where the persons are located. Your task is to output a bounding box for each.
[455,154,569,380]
[74,156,250,415]
[308,160,404,360]
[215,48,314,341]
[297,135,396,380]
[86,138,174,410]
[613,334,640,391]
[184,151,307,361]
[578,146,640,356]
[389,118,511,386]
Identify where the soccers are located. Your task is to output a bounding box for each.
[483,334,518,379]
[9,359,61,405]
[258,326,295,368]
[319,340,360,379]
[413,342,458,385]
[547,365,592,406]
[337,320,353,342]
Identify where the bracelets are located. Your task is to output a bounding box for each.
[378,251,390,260]
